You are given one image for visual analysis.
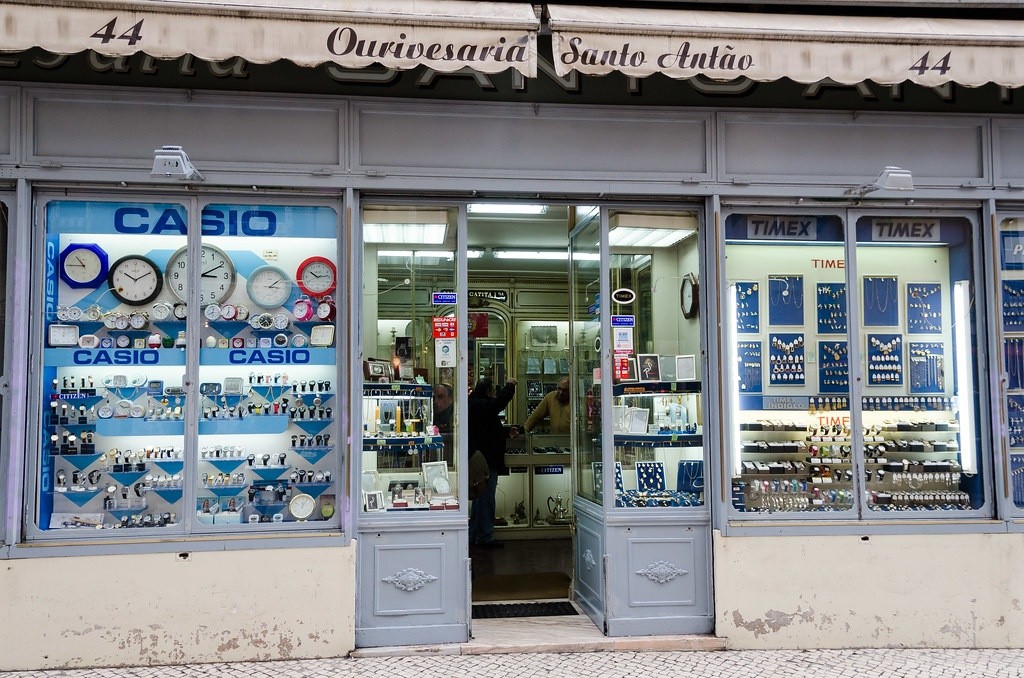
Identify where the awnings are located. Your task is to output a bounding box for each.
[0,0,1024,107]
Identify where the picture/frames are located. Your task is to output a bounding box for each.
[620,358,638,382]
[364,491,379,509]
[676,354,696,379]
[422,457,447,493]
[612,405,629,435]
[629,407,650,435]
[378,489,385,507]
[637,353,660,381]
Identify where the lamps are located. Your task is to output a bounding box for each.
[147,146,205,186]
[847,161,917,209]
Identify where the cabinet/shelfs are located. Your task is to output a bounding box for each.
[577,375,707,513]
[986,225,1024,521]
[359,381,456,517]
[472,440,628,537]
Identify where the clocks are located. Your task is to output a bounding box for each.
[434,472,450,496]
[49,243,335,349]
[98,399,145,419]
[289,493,316,523]
[679,272,698,320]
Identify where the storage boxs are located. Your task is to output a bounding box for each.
[736,408,970,515]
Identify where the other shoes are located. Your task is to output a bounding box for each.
[477,540,504,548]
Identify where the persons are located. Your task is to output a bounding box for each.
[468,376,519,549]
[521,375,573,436]
[431,381,455,468]
